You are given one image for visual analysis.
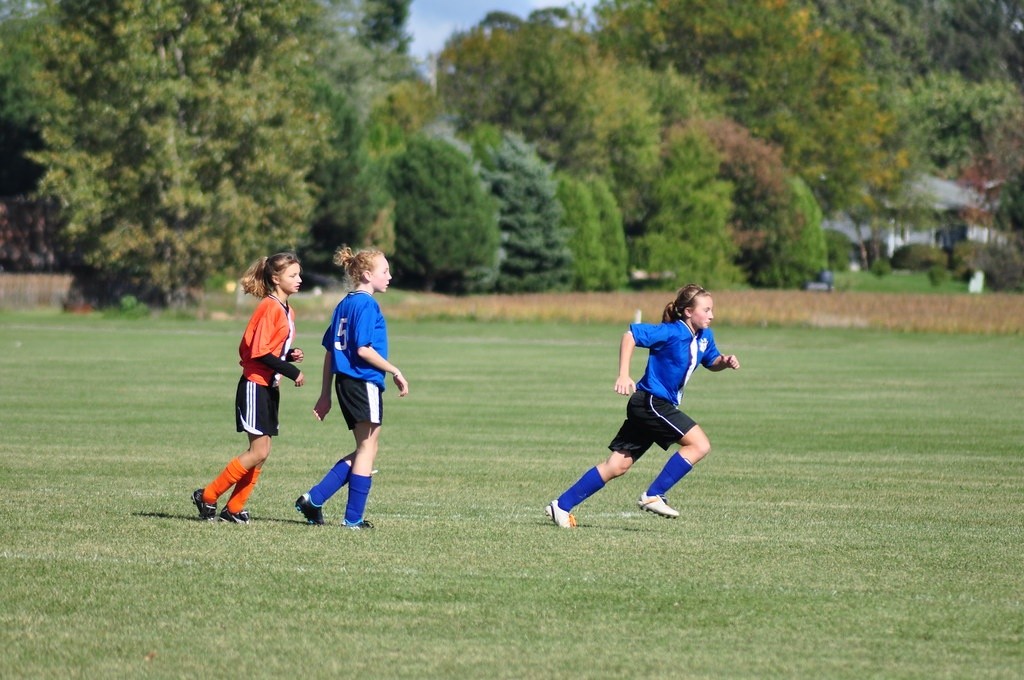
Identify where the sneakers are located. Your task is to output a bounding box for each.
[295,492,325,525]
[191,489,217,521]
[544,500,576,528]
[638,491,679,519]
[218,505,250,525]
[341,518,374,530]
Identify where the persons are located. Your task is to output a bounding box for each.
[545,283,741,532]
[190,253,304,526]
[296,247,409,529]
